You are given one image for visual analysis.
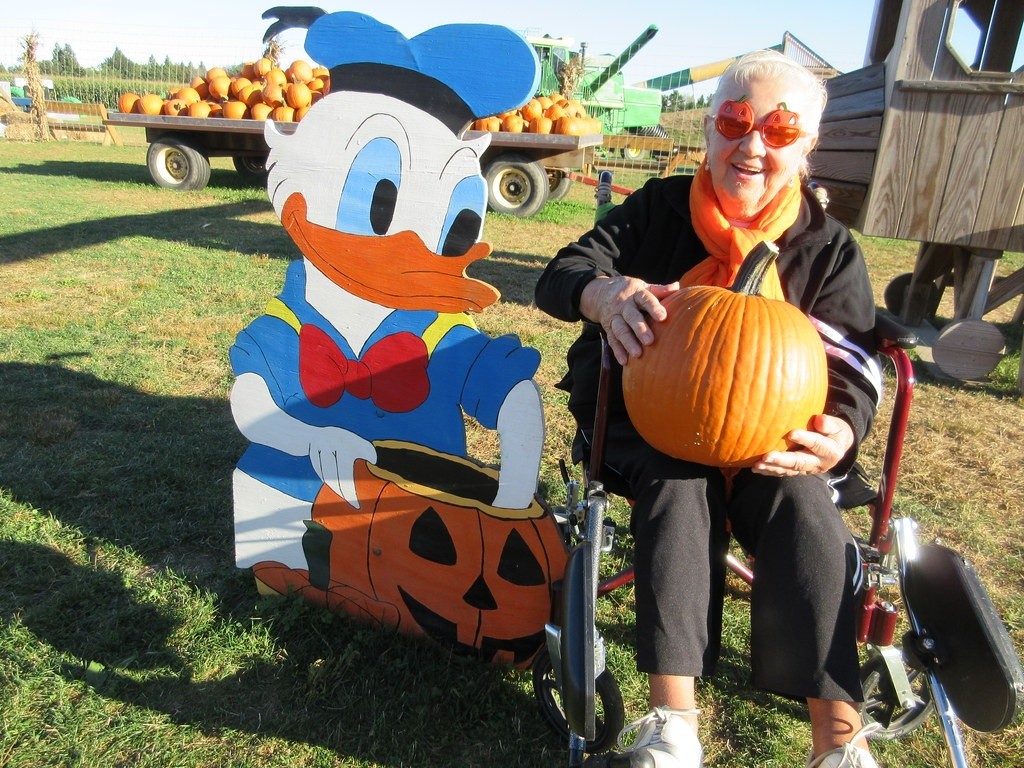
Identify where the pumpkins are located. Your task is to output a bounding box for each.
[466,93,601,135]
[622,240,828,467]
[118,58,331,123]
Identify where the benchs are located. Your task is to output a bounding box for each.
[588,134,692,178]
[44,99,123,148]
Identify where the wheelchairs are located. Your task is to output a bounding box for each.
[531,168,1023,767]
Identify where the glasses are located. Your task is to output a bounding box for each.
[711,94,817,149]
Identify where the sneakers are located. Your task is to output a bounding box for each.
[805,721,880,768]
[617,705,703,768]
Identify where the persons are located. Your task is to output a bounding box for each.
[534,50,886,768]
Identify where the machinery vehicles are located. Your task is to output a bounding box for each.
[523,25,784,160]
